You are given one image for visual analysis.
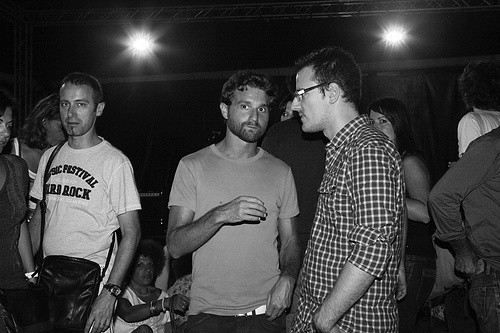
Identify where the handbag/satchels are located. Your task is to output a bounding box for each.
[170,294,187,333]
[30,255,100,329]
[0,293,23,333]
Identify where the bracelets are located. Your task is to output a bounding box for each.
[162,297,166,312]
[150,301,153,316]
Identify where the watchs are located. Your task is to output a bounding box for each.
[24,271,39,279]
[103,283,124,298]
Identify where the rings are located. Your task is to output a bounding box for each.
[182,305,185,309]
[184,302,186,304]
[100,323,105,326]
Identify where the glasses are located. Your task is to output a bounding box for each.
[290,80,336,99]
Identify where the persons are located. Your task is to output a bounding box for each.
[290,47,411,333]
[4,93,68,222]
[0,97,40,288]
[428,58,500,333]
[27,72,143,333]
[369,98,431,333]
[168,70,301,333]
[276,92,296,123]
[106,239,190,333]
